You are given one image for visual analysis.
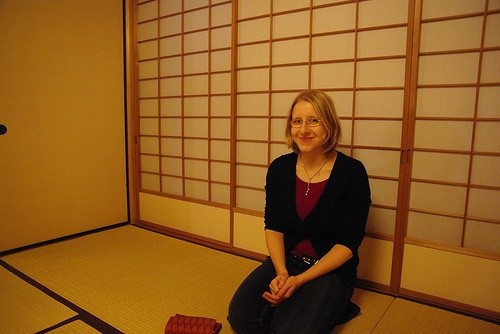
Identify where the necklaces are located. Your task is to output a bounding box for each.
[300,153,329,195]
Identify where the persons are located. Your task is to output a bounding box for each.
[228,89,372,334]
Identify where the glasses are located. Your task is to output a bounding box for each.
[290,119,322,128]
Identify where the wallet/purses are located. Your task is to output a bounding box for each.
[164,314,222,334]
